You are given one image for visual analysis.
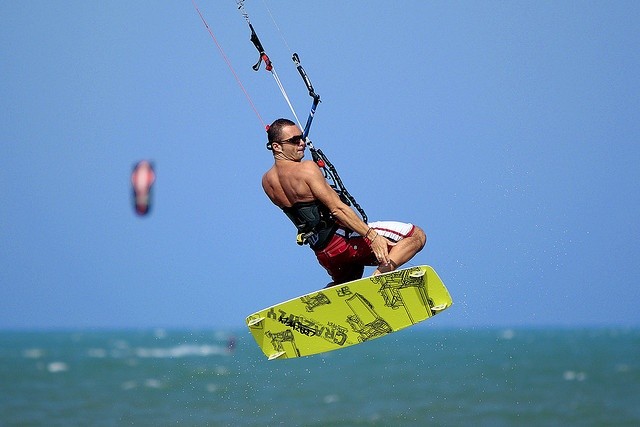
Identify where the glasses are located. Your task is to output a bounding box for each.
[274,134,305,145]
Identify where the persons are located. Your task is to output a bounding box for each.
[259,118,427,289]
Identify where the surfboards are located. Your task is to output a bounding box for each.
[245,265,454,360]
